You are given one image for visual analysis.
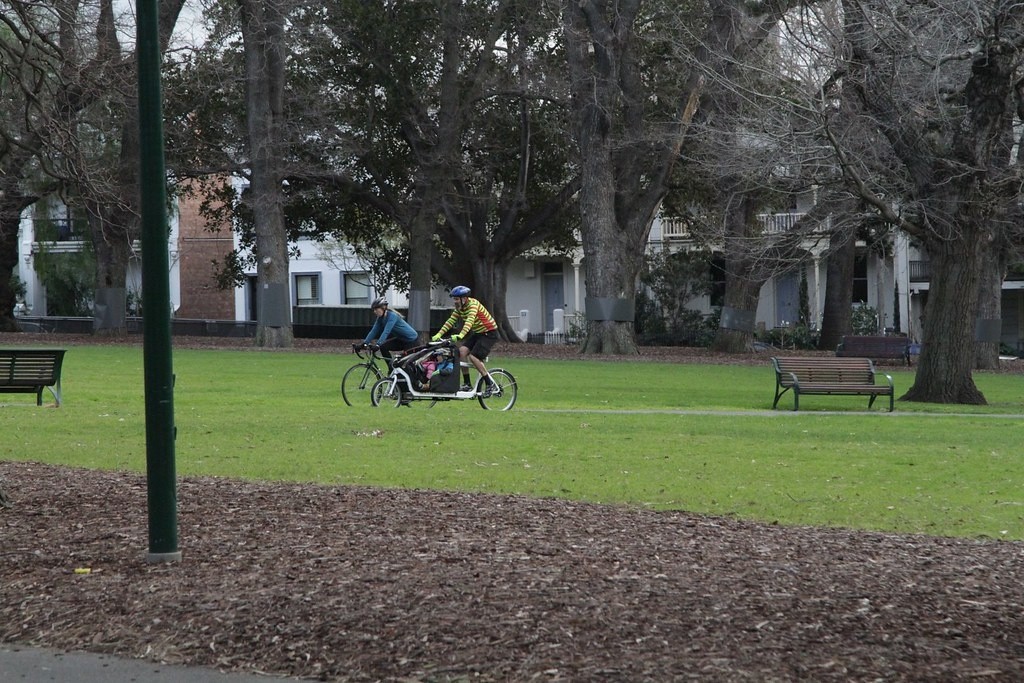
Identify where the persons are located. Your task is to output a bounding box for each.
[415,348,453,388]
[358,298,420,376]
[432,286,500,399]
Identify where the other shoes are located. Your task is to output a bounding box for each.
[483,382,494,397]
[459,383,472,391]
[417,380,426,391]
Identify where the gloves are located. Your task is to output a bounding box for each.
[432,369,440,376]
[371,344,380,352]
[432,333,442,341]
[451,333,464,342]
[359,342,367,350]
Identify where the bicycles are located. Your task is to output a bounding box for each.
[372,337,519,412]
[340,342,439,412]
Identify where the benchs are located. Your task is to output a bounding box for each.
[835,335,913,366]
[0,348,68,407]
[769,356,894,413]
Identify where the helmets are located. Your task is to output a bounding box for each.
[372,297,388,308]
[434,349,451,356]
[450,286,471,297]
